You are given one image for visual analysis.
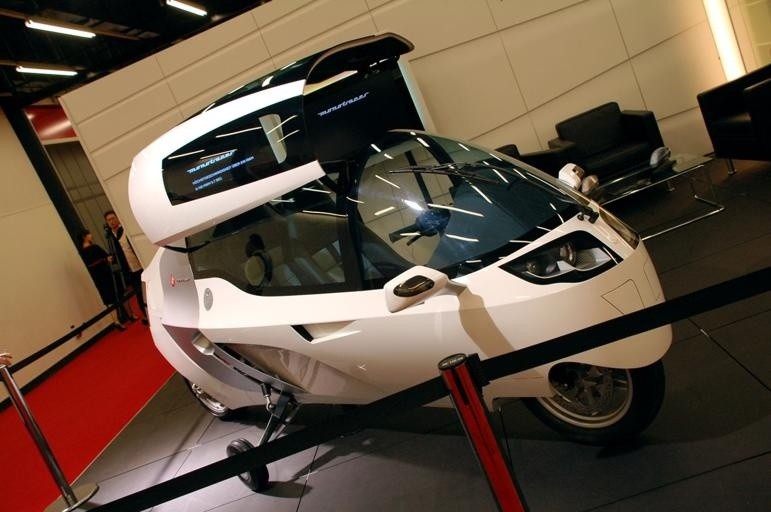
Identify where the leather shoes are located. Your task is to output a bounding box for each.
[142,318,148,325]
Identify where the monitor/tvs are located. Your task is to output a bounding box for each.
[280,55,426,168]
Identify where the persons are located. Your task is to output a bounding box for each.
[77,228,140,331]
[104,210,149,326]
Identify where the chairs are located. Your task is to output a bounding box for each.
[548,101,674,191]
[697,63,771,175]
[448,143,567,201]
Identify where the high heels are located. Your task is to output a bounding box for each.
[114,324,126,331]
[129,315,138,323]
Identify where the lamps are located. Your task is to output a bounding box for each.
[166,0,207,16]
[25,18,96,38]
[16,64,78,76]
